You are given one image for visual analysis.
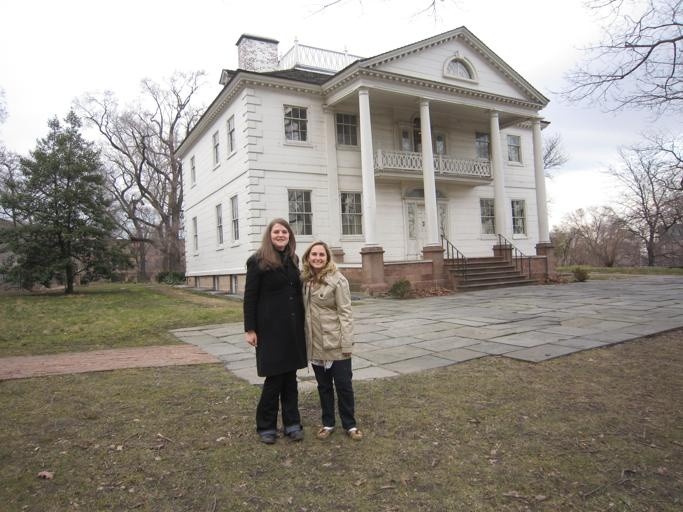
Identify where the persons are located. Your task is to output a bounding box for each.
[243,218,309,446]
[301,241,362,439]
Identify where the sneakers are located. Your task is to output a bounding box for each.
[261,434,275,444]
[345,428,363,441]
[317,425,336,440]
[288,430,304,441]
